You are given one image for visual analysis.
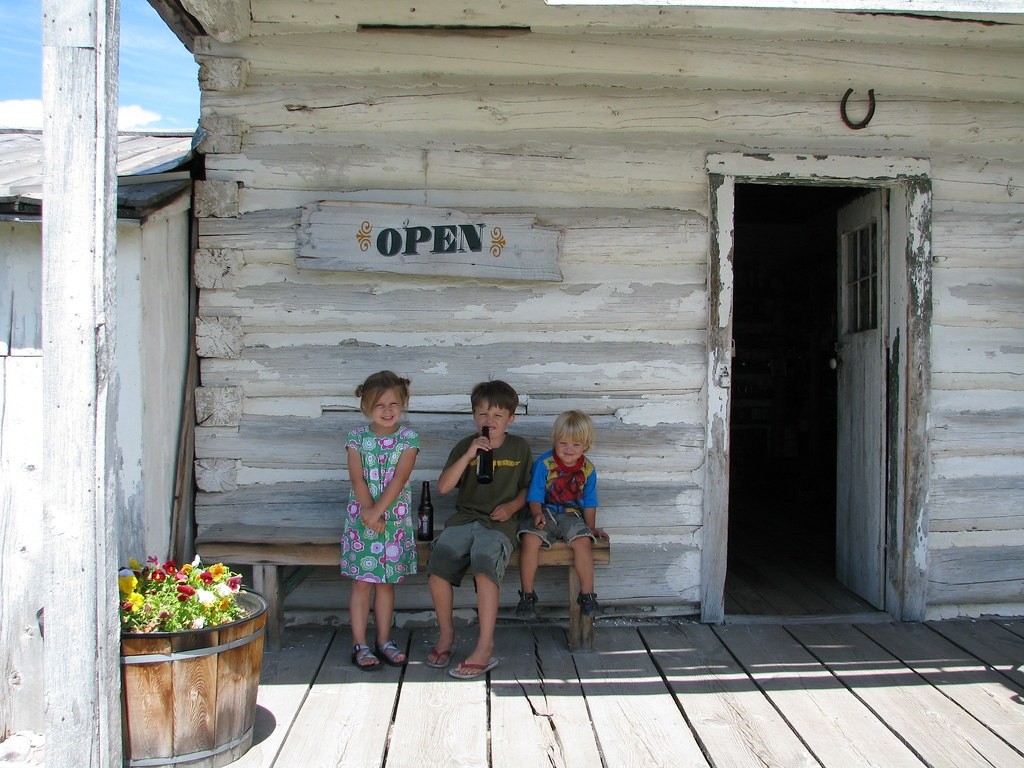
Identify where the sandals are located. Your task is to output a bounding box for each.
[373,639,409,667]
[351,645,384,669]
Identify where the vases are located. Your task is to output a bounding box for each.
[120,587,270,768]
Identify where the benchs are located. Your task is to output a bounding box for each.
[194,523,610,652]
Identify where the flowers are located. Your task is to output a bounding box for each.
[119,554,246,632]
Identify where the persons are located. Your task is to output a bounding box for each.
[340,371,421,671]
[516,410,608,619]
[426,381,533,679]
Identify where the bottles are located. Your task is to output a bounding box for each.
[476,426,493,484]
[419,481,434,541]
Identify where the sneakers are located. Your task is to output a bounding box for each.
[515,589,538,618]
[577,592,600,618]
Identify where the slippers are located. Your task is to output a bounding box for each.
[426,640,457,667]
[448,655,498,679]
